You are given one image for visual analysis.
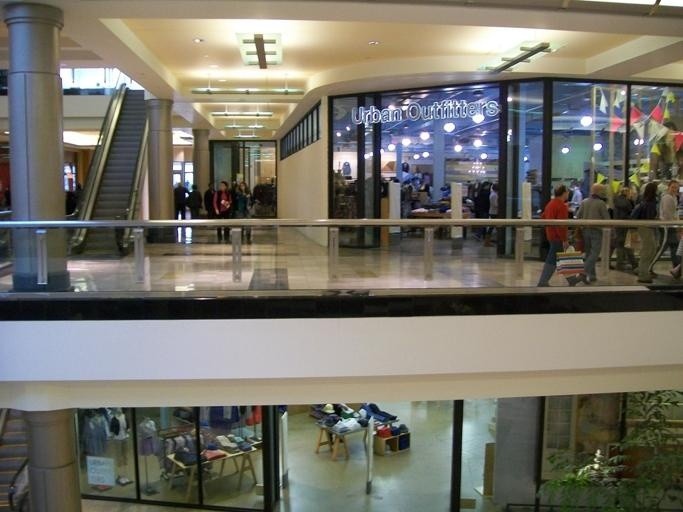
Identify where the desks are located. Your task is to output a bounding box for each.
[314,413,400,463]
[164,440,258,501]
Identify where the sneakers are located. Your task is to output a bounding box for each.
[568,275,597,287]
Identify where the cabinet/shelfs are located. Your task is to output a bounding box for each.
[372,431,411,458]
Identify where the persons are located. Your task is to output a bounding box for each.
[138,414,157,457]
[82,410,107,457]
[111,407,129,478]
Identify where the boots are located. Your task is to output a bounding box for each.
[482,234,496,248]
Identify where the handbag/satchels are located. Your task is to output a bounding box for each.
[623,227,645,250]
[555,245,584,274]
